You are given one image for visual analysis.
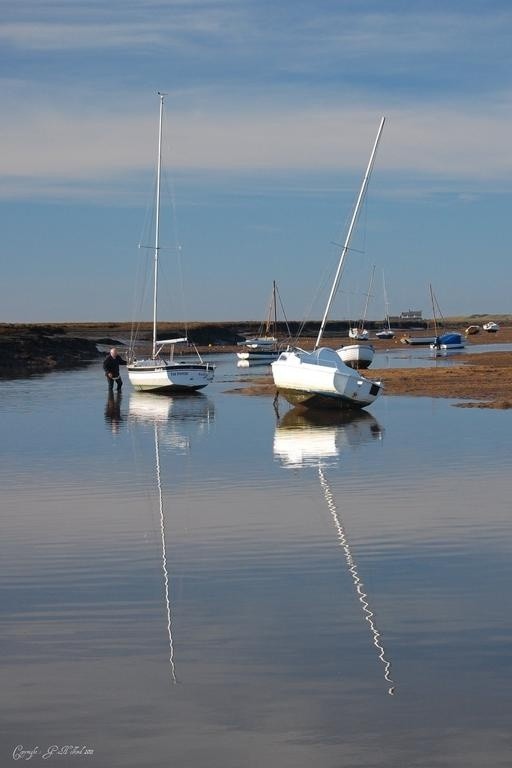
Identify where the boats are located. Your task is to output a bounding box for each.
[335,344,375,370]
[401,334,436,345]
[483,321,499,332]
[465,325,479,335]
[243,335,279,349]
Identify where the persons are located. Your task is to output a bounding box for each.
[102,347,129,392]
[436,336,443,349]
[104,392,124,436]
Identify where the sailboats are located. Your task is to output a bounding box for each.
[271,117,391,411]
[235,279,294,362]
[122,90,216,390]
[428,284,464,350]
[269,399,396,701]
[128,391,217,684]
[347,263,378,342]
[374,270,393,339]
[237,360,274,374]
